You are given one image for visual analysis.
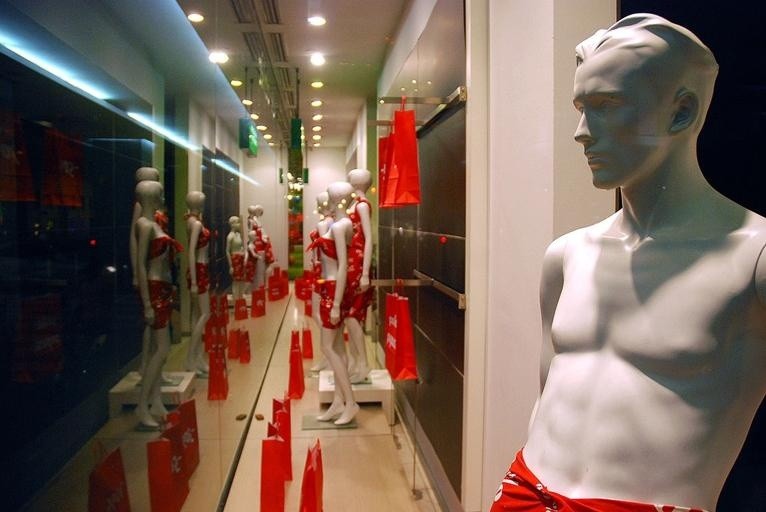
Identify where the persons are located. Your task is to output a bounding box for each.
[225,204,274,299]
[130,167,173,382]
[308,168,376,424]
[182,191,211,373]
[490,12,766,512]
[133,181,183,427]
